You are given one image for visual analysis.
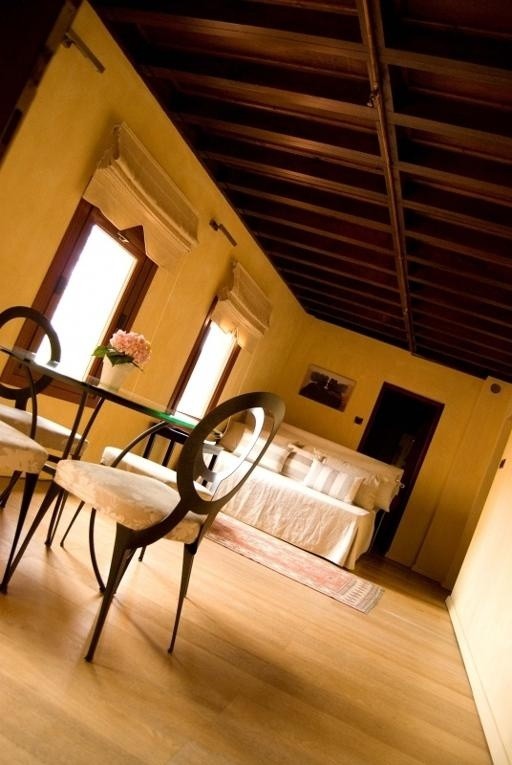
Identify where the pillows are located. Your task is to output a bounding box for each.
[252,427,301,452]
[281,444,324,482]
[302,460,365,505]
[233,427,294,473]
[320,456,381,510]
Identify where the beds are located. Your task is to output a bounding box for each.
[195,421,404,570]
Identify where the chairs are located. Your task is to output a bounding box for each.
[1,418,48,593]
[60,406,266,596]
[0,306,89,547]
[0,392,285,660]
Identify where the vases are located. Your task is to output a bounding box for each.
[96,355,133,394]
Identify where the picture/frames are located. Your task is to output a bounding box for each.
[299,363,357,413]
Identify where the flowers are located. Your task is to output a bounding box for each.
[91,329,152,367]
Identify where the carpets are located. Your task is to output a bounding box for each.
[206,511,386,614]
